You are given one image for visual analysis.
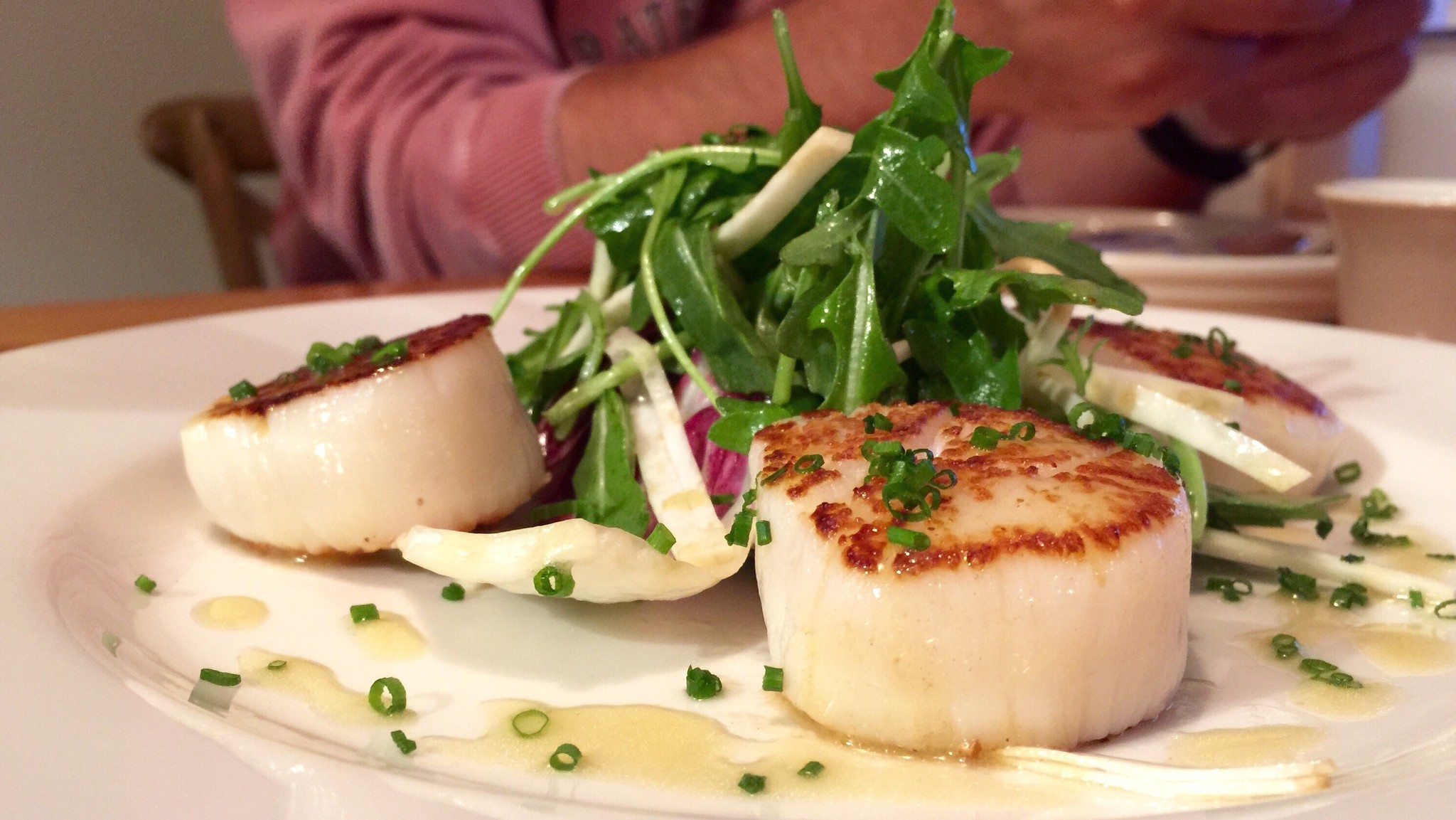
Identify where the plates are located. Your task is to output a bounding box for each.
[0,283,1456,820]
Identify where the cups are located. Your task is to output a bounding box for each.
[1315,177,1456,344]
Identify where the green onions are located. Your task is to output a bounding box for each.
[127,340,1456,796]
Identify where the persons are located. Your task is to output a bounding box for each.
[224,0,1432,285]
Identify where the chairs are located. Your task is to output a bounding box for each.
[142,88,283,295]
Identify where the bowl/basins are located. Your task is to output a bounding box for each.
[992,204,1338,320]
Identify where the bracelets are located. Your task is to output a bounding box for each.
[1137,112,1246,183]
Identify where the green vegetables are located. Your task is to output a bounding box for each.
[480,0,1354,556]
